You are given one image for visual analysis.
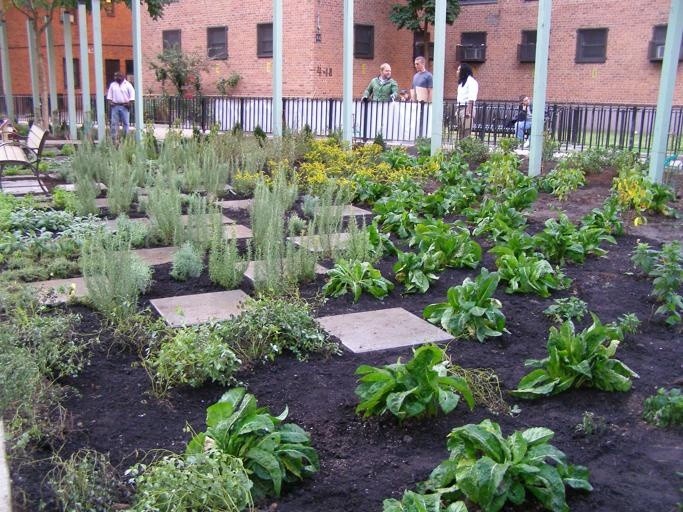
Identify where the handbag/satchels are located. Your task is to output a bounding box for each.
[462,114,473,129]
[503,114,518,128]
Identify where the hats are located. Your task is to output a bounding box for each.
[400,89,407,95]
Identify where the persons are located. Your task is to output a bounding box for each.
[513,94,532,147]
[454,62,478,138]
[410,56,431,103]
[360,62,398,102]
[106,70,135,144]
[399,89,411,102]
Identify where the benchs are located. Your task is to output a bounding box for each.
[449,106,550,139]
[0,122,52,198]
